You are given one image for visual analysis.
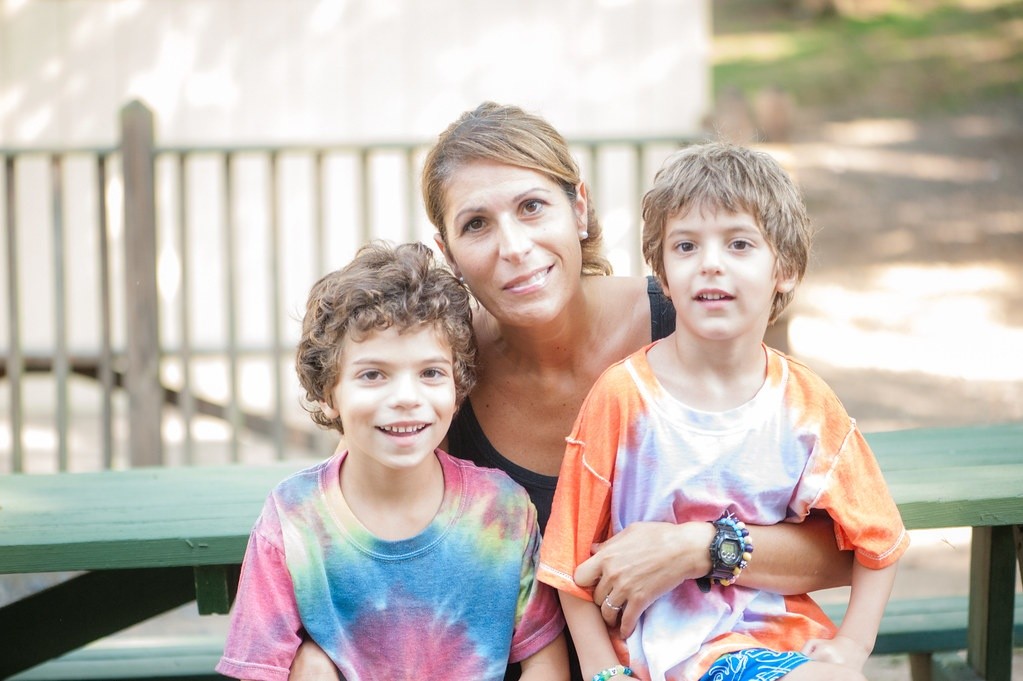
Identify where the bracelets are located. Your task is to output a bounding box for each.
[712,511,752,587]
[590,665,631,681]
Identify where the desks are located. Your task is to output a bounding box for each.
[0,411,1023,681]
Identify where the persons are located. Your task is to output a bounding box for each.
[536,142,910,681]
[213,241,573,681]
[288,98,854,681]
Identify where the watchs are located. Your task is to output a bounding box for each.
[706,515,740,585]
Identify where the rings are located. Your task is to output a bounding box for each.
[605,597,624,610]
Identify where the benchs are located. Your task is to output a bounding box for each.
[0,588,1023,681]
